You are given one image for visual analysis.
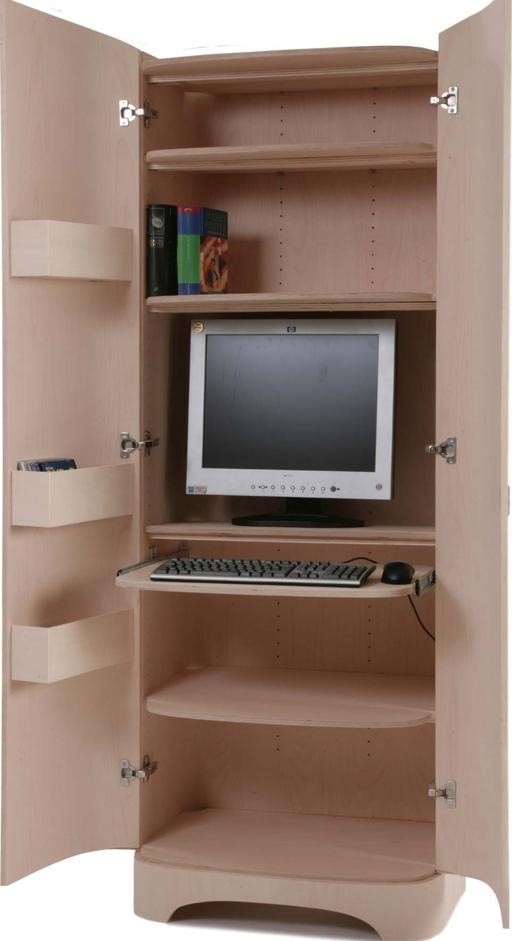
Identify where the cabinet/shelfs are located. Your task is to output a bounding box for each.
[0,0,511,934]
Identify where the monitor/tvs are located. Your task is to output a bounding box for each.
[185,318,398,529]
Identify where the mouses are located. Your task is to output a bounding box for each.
[381,559,417,584]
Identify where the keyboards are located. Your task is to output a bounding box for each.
[149,555,376,587]
